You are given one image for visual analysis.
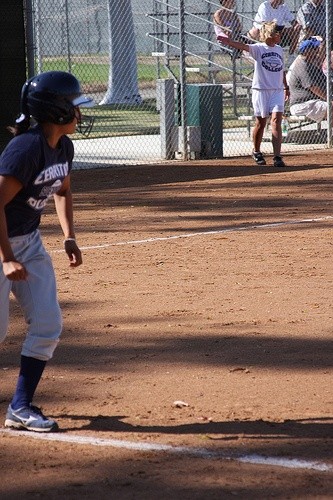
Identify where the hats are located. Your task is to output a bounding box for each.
[299,40,320,53]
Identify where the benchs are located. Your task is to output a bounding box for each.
[238,115,326,139]
[152,51,233,84]
[222,83,253,115]
[186,65,255,84]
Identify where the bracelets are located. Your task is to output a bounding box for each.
[285,86,289,90]
[63,238,75,243]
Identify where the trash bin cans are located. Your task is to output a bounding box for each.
[175,82,225,159]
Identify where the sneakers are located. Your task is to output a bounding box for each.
[5,404,58,433]
[252,149,266,165]
[273,156,285,166]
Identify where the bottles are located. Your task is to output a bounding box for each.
[281,116,288,137]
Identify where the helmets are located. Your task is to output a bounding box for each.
[26,71,87,123]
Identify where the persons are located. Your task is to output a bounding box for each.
[286,39,333,123]
[296,0,333,50]
[0,71,83,432]
[214,0,260,65]
[217,24,290,166]
[253,0,303,55]
